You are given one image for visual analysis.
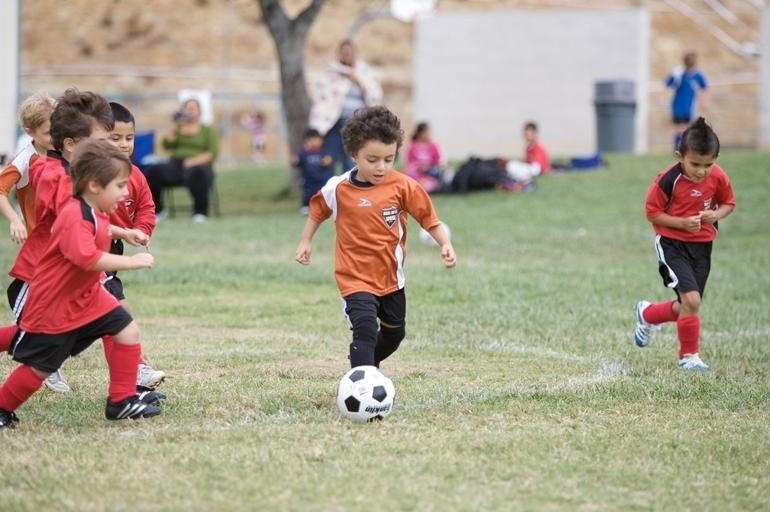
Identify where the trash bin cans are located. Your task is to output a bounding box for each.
[594,80,636,152]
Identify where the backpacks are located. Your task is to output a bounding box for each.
[451,157,500,194]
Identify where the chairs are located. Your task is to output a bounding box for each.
[167,176,219,216]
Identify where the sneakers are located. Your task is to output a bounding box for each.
[635,300,653,348]
[678,352,710,374]
[0,408,19,429]
[153,206,210,224]
[104,396,159,419]
[137,364,167,389]
[135,384,165,402]
[44,368,70,393]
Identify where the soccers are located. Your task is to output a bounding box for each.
[337,365,396,419]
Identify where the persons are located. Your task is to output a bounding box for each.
[294,105,455,367]
[142,99,217,221]
[0,87,163,426]
[506,123,546,191]
[247,113,265,162]
[292,39,380,212]
[633,118,736,372]
[665,52,708,149]
[405,123,453,192]
